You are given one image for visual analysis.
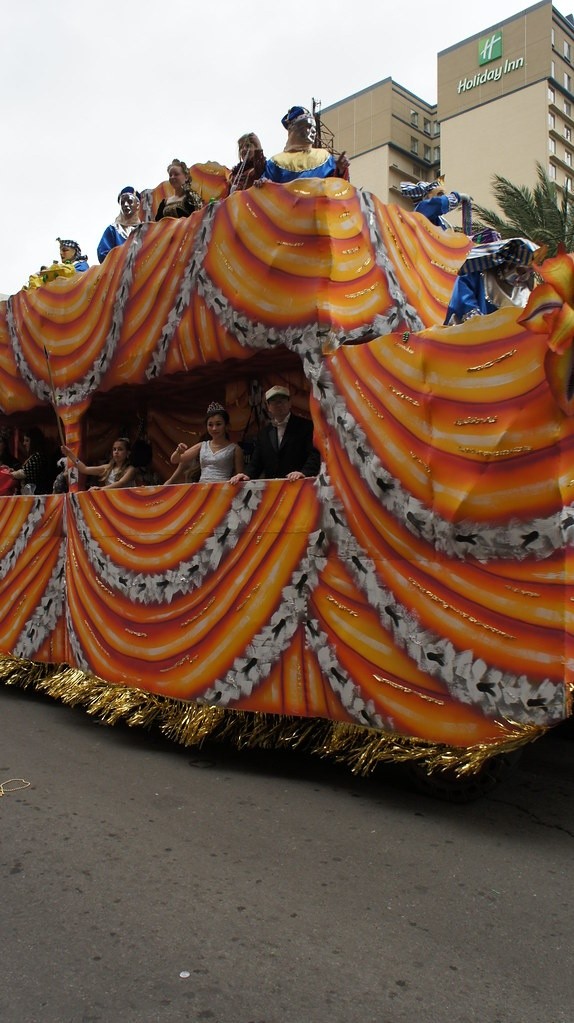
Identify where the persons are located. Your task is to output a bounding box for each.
[444,229,542,326]
[226,132,267,197]
[254,106,351,188]
[227,385,320,486]
[61,438,135,491]
[155,159,203,221]
[399,181,475,233]
[55,237,89,272]
[97,187,144,264]
[171,402,243,483]
[0,422,69,496]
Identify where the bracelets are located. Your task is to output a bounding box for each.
[72,458,79,466]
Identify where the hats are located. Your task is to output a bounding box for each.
[401,174,445,198]
[264,385,290,403]
[118,186,141,202]
[281,106,312,129]
